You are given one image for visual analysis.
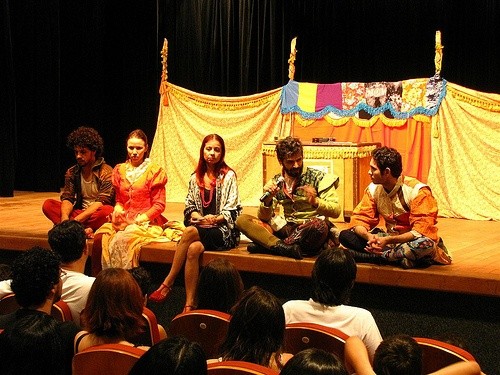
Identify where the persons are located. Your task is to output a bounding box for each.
[0,247,80,360]
[198,258,243,313]
[6,314,63,375]
[91,130,185,277]
[345,335,481,375]
[42,127,113,238]
[339,146,451,268]
[128,267,167,339]
[206,287,293,371]
[73,268,151,354]
[279,348,347,375]
[237,136,342,260]
[149,133,243,311]
[128,335,208,375]
[0,221,96,329]
[282,249,383,359]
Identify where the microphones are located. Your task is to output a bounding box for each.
[260,177,285,201]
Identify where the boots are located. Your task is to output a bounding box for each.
[347,249,380,263]
[247,242,267,253]
[271,240,302,260]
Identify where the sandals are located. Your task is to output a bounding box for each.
[148,284,171,304]
[182,301,197,313]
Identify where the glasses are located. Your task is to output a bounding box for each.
[59,269,67,277]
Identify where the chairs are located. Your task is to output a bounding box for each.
[171,310,233,360]
[72,343,147,375]
[411,336,476,375]
[284,323,350,361]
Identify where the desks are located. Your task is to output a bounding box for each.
[262,141,382,224]
